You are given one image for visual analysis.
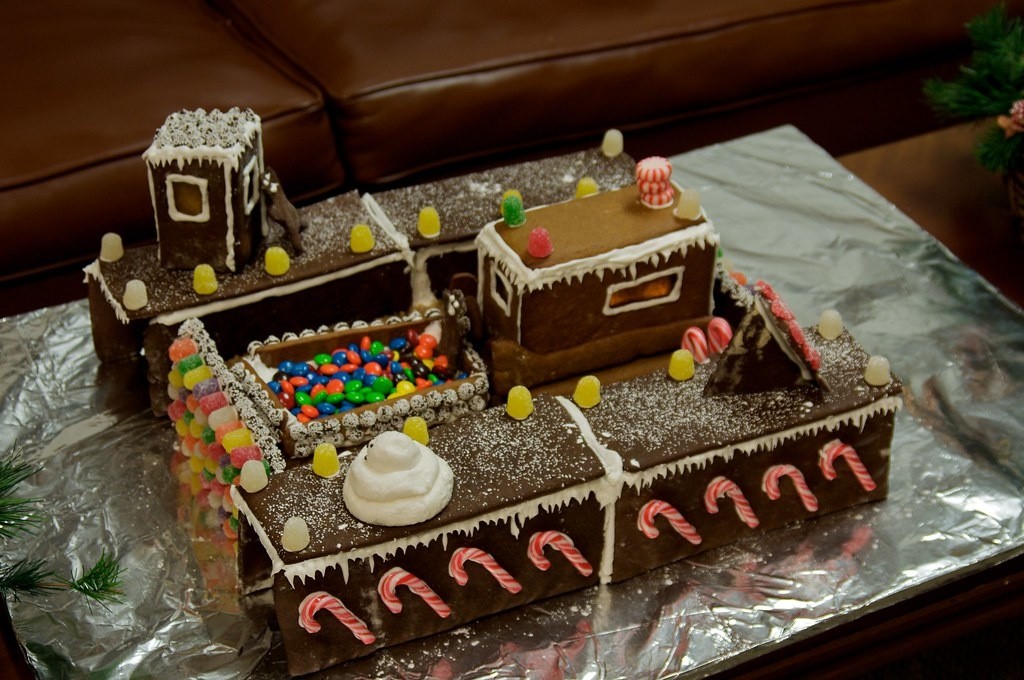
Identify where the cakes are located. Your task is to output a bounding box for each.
[82,107,900,677]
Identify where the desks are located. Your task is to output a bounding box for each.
[707,123,1024,680]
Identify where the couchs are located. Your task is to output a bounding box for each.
[0,0,1024,294]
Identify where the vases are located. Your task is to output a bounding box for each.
[1009,173,1024,217]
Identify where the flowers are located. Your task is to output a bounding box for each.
[921,2,1024,180]
